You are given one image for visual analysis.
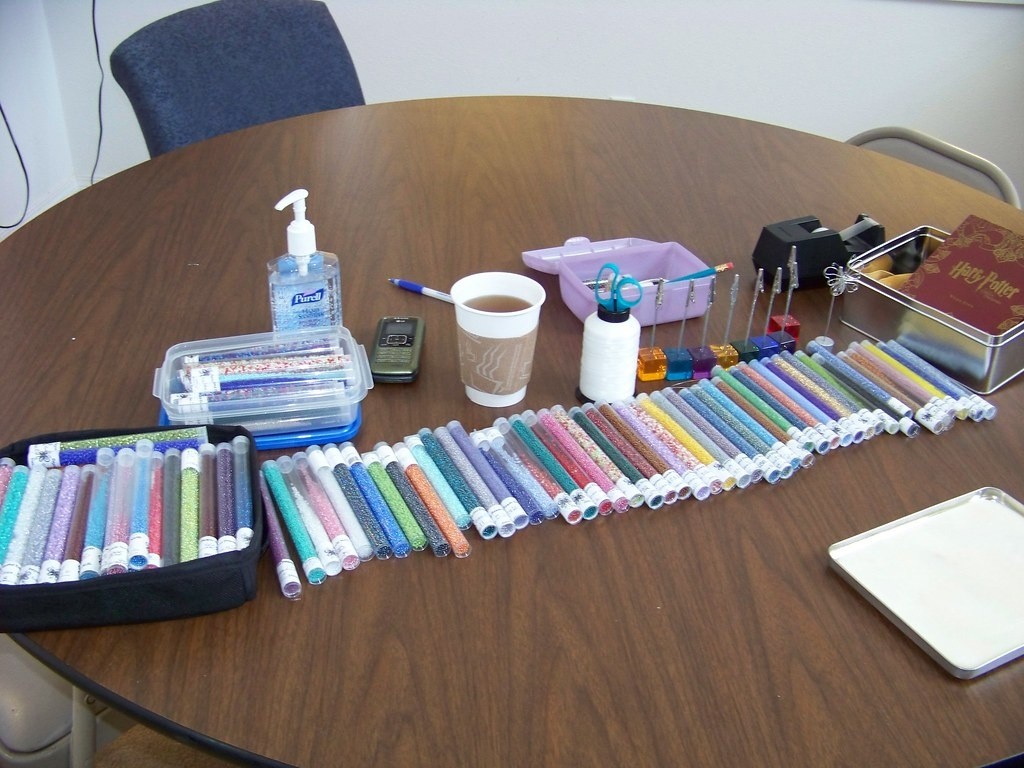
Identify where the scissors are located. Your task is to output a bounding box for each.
[594,261,645,315]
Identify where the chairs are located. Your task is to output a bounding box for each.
[114,0,367,156]
[844,126,1020,211]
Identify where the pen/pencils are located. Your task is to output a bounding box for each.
[581,261,736,288]
[386,276,456,305]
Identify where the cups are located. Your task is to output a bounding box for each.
[450,272,546,407]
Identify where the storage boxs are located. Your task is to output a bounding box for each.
[151,325,376,437]
[519,236,716,327]
[0,424,262,633]
[840,224,1024,394]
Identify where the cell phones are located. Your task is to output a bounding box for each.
[370,316,425,382]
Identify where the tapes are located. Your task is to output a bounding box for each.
[809,217,880,242]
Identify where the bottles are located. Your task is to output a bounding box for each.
[578,299,641,404]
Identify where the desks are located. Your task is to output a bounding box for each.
[1,94,1024,768]
